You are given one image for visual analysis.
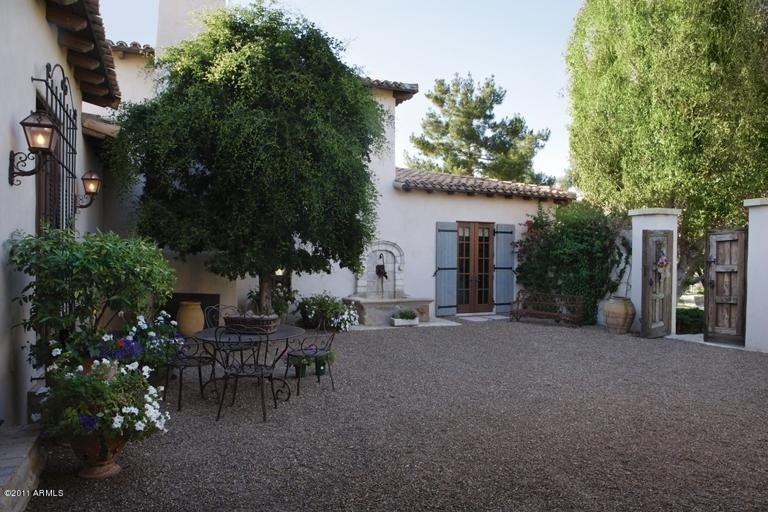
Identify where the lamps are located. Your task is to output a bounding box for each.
[9,110,59,186]
[75,170,104,208]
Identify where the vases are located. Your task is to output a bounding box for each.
[71,435,121,480]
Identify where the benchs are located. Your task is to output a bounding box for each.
[511,288,582,325]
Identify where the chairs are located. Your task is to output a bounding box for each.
[163,304,336,423]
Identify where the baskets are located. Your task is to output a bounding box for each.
[224,299,279,334]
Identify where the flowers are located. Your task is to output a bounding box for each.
[30,311,185,438]
[296,289,359,333]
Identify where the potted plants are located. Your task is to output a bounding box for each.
[390,310,420,326]
